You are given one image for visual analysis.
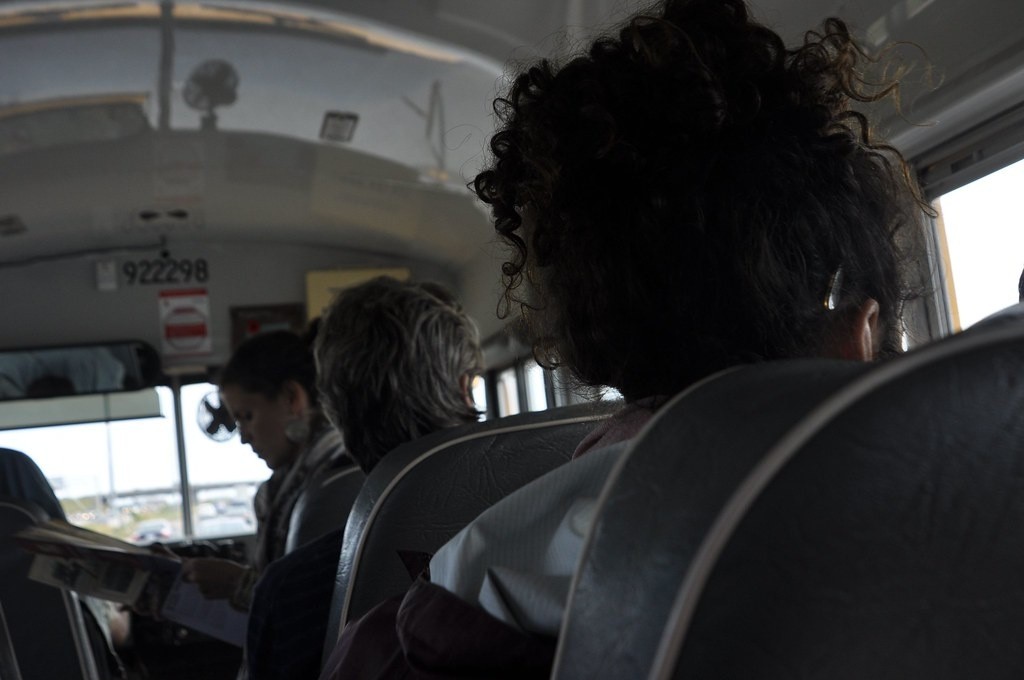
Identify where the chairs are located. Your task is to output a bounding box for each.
[0,502,100,680]
[283,318,1024,679]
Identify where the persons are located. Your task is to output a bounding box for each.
[245,275,483,680]
[320,0,928,680]
[0,448,129,647]
[146,331,366,680]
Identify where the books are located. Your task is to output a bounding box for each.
[10,516,187,618]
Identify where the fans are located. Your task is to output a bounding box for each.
[198,391,237,442]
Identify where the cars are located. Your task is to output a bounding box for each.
[130,520,175,544]
